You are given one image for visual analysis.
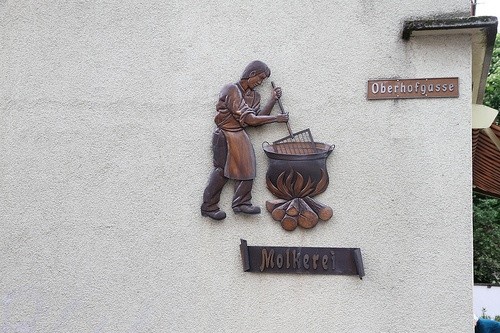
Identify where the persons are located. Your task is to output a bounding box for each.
[199,58,290,221]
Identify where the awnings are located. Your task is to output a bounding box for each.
[471,123,500,199]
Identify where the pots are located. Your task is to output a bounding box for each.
[260,141,335,197]
